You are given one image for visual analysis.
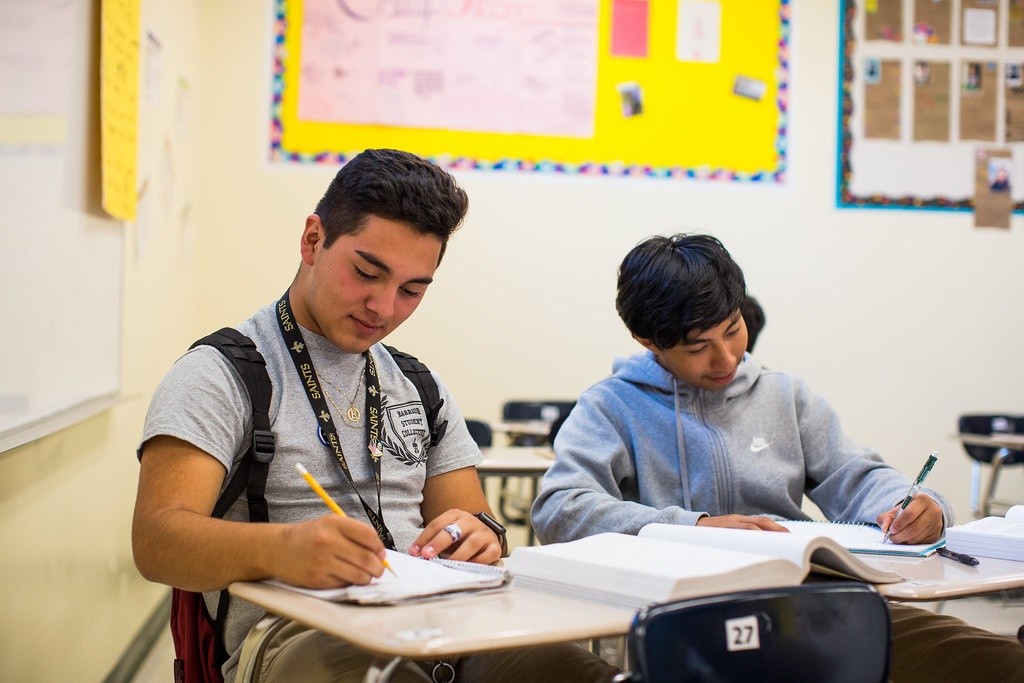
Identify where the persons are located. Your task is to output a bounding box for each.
[132,149,625,683]
[990,171,1010,189]
[528,236,954,683]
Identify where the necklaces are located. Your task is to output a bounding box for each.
[317,375,366,428]
[316,368,366,421]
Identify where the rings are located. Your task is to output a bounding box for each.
[443,525,461,543]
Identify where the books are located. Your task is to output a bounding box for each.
[263,548,511,605]
[777,519,945,558]
[505,523,906,613]
[946,505,1024,562]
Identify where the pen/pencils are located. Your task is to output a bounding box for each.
[293,460,402,582]
[882,449,940,546]
[935,547,980,567]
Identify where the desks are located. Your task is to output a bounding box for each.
[476,444,557,545]
[954,432,1024,517]
[230,548,1024,683]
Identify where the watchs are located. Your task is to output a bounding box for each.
[474,512,508,556]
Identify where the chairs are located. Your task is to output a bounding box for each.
[467,400,576,525]
[629,581,892,683]
[957,414,1024,513]
